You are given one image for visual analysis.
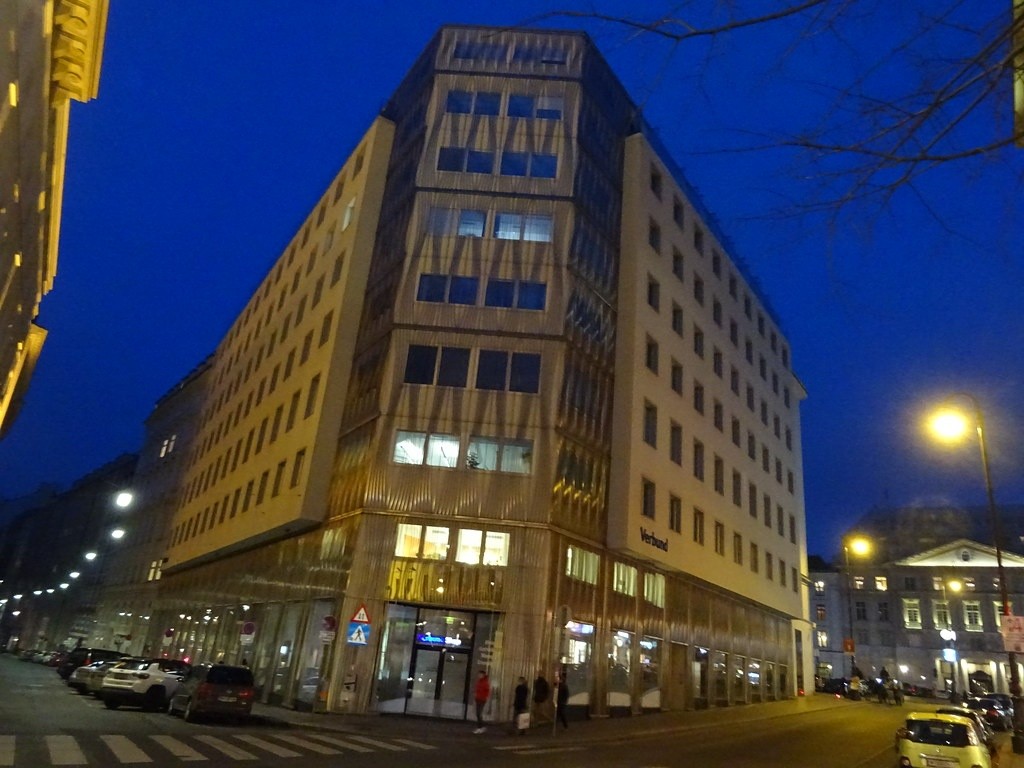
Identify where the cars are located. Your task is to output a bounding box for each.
[895,713,991,768]
[68,660,122,695]
[962,692,1015,733]
[19,648,69,668]
[935,707,991,746]
[843,678,918,696]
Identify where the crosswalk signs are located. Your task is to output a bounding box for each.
[347,622,372,647]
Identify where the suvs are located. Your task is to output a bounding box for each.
[57,648,131,679]
[102,655,193,710]
[167,662,256,724]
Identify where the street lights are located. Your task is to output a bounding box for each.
[938,579,963,702]
[923,393,1024,755]
[841,536,871,671]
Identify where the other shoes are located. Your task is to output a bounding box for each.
[473,727,486,733]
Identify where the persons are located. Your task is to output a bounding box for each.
[472,670,489,734]
[835,666,905,706]
[509,677,530,736]
[532,671,569,734]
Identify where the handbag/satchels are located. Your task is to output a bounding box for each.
[517,713,530,729]
